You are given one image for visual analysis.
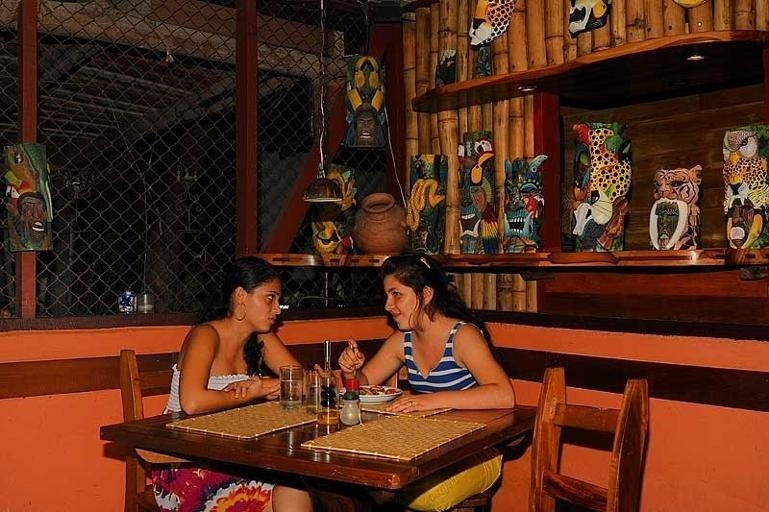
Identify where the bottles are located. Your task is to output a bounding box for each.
[340,391,361,425]
[317,340,339,424]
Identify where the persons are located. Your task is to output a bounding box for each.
[339,251,515,511]
[150,256,307,512]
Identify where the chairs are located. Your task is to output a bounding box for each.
[386,367,491,511]
[529,368,650,511]
[120,348,180,512]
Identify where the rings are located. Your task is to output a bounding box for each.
[408,401,414,407]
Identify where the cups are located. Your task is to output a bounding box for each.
[279,365,304,411]
[138,294,154,314]
[304,370,321,416]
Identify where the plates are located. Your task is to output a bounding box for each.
[339,385,403,404]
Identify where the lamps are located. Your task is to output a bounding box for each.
[303,1,343,202]
